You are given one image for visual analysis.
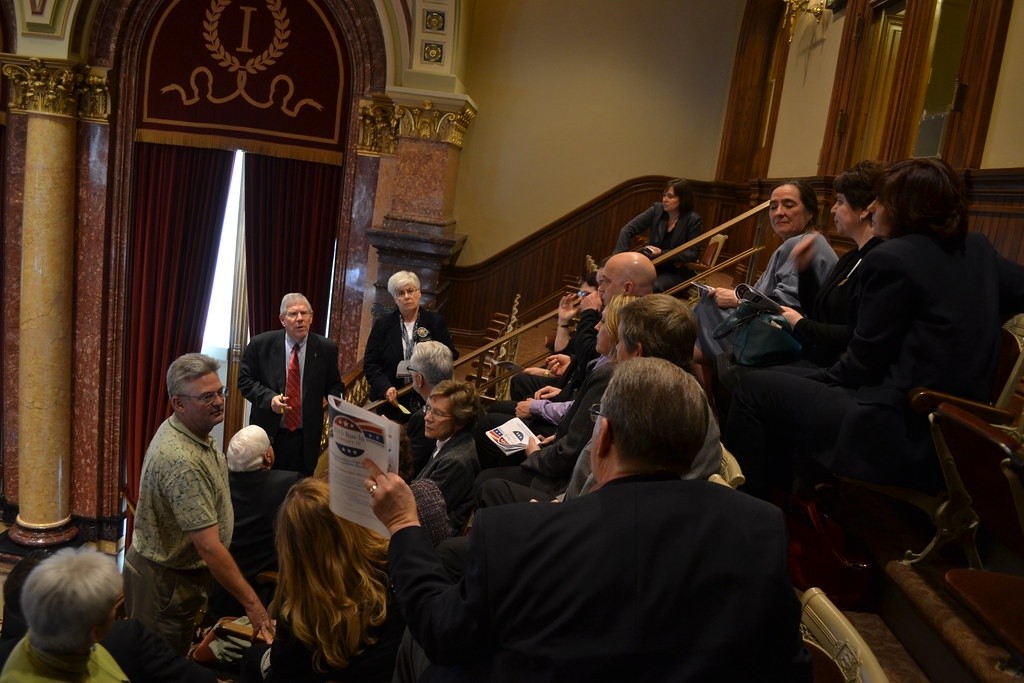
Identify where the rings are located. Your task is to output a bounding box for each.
[368,484,378,494]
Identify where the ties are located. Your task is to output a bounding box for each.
[283,343,303,432]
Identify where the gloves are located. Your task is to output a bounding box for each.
[209,627,252,663]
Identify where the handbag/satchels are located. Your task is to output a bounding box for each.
[712,302,803,368]
[784,493,879,611]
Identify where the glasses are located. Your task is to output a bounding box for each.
[396,288,420,297]
[578,291,591,296]
[422,405,454,417]
[175,386,230,402]
[588,404,615,444]
[406,364,419,373]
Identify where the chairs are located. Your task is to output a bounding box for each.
[214,225,1024,683]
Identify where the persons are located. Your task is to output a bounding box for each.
[122,352,276,652]
[0,157,1024,683]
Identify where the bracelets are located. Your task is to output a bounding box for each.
[557,320,570,328]
[544,369,549,377]
